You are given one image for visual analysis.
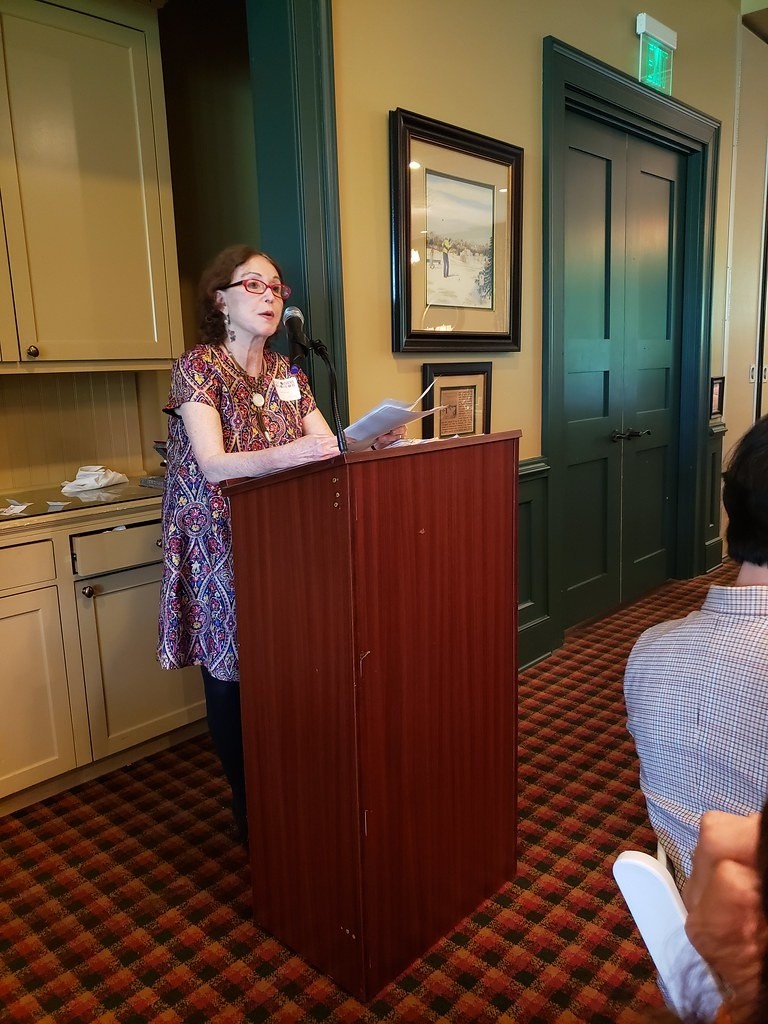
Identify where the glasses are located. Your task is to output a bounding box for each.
[220,278,292,299]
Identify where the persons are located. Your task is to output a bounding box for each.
[442,238,452,278]
[155,245,407,839]
[623,414,768,1024]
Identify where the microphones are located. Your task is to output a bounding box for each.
[283,306,304,374]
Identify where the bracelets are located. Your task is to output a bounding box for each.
[371,445,376,450]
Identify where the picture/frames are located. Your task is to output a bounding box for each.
[389,106,522,352]
[421,361,494,439]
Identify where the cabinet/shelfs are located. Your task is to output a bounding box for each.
[0,504,207,795]
[709,376,726,416]
[0,0,183,376]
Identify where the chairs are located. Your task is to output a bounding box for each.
[614,835,726,1019]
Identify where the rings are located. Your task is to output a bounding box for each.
[400,435,401,438]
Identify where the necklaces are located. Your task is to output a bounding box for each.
[222,341,273,443]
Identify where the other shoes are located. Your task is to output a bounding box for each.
[237,827,248,853]
